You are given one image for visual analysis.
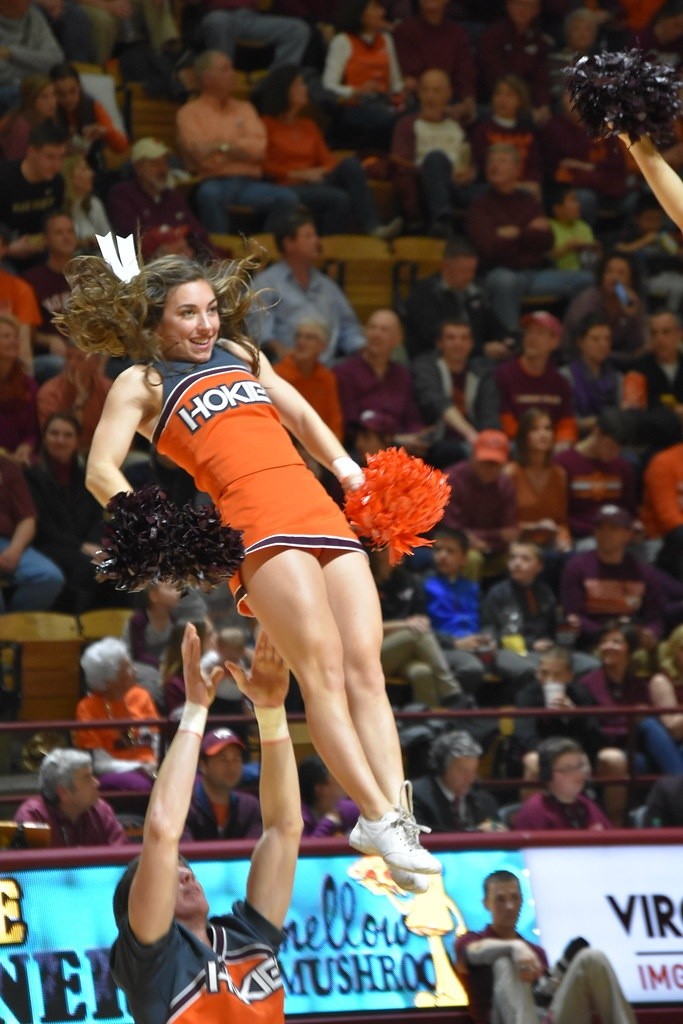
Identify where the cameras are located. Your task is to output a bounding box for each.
[532,937,589,1008]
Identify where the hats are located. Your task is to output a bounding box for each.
[474,429,509,465]
[202,728,246,757]
[132,137,166,160]
[593,503,630,529]
[432,730,482,769]
[525,309,563,334]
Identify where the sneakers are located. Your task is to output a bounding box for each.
[348,780,441,893]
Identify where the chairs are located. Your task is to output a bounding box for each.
[74,56,271,163]
[200,234,476,329]
[0,607,136,724]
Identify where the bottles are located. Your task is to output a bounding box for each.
[476,637,495,666]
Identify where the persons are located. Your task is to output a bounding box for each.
[0,0,683,1024]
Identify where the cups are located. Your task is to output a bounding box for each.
[502,605,522,635]
[541,682,565,708]
